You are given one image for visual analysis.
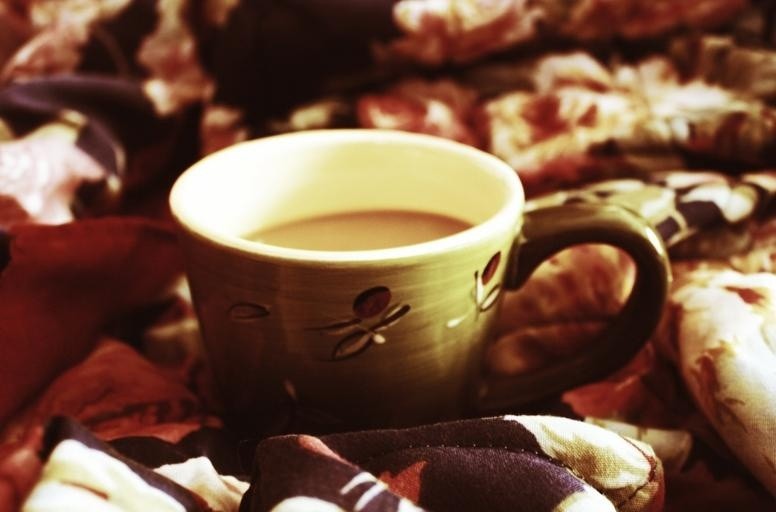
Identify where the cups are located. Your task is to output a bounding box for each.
[166,126,679,455]
